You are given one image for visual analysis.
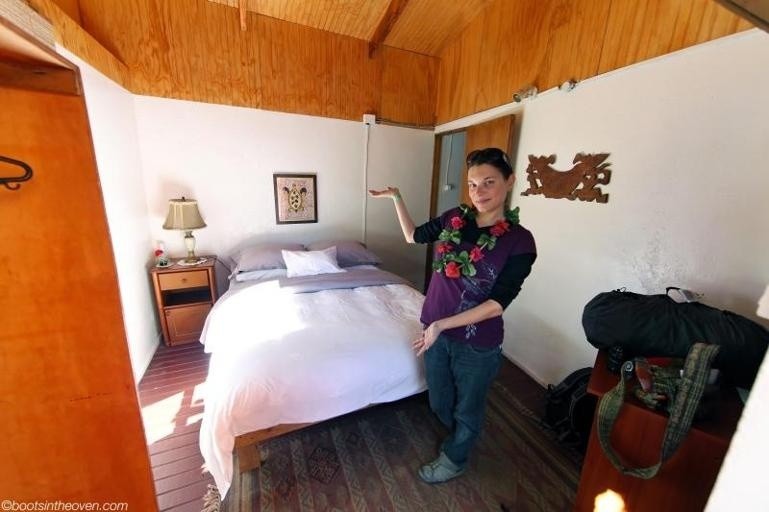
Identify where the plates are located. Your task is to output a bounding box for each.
[178,257,207,266]
[156,262,174,267]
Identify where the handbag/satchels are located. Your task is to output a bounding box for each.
[631,357,719,420]
[582,287,768,390]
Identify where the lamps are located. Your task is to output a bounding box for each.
[511,86,538,104]
[162,197,207,266]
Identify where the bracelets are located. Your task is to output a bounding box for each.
[390,193,402,201]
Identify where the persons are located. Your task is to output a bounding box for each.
[367,146,538,485]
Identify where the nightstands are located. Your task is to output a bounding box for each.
[152,255,219,347]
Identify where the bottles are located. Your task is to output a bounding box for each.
[159,240,169,267]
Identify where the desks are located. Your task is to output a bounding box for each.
[573,348,746,511]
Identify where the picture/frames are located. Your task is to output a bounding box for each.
[273,173,318,225]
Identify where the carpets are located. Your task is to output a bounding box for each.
[199,378,581,510]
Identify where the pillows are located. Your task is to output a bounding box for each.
[220,239,385,281]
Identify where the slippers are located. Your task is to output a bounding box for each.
[418,458,466,484]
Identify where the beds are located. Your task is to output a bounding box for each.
[205,263,442,472]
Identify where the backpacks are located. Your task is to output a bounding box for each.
[545,367,598,435]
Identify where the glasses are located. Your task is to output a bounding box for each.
[466,148,513,173]
